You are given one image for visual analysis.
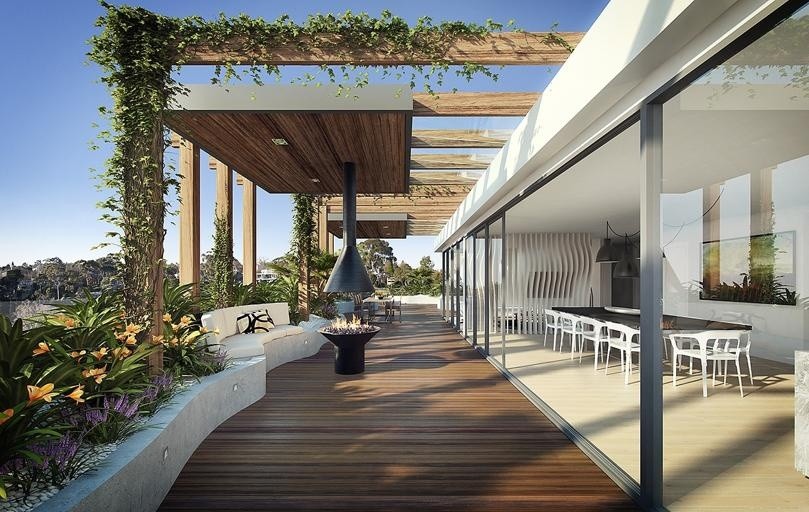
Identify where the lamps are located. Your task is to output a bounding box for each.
[636,245,667,259]
[596,220,621,264]
[613,233,640,278]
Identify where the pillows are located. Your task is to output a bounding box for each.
[200,302,290,352]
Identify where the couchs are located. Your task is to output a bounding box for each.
[198,302,321,416]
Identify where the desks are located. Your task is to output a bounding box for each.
[385,300,402,323]
[552,306,753,385]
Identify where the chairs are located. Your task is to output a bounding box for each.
[543,309,754,398]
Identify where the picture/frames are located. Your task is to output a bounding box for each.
[699,231,797,306]
